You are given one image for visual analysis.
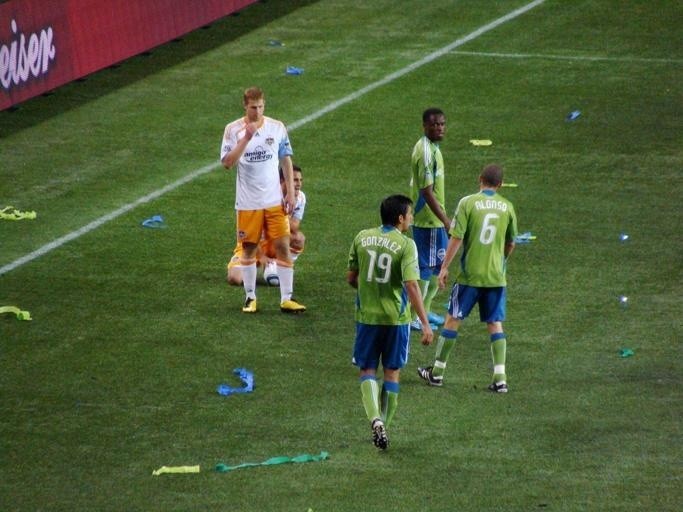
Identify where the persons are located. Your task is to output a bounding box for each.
[348,195,433,449]
[417,165,518,393]
[221,88,307,313]
[228,164,307,286]
[407,109,452,332]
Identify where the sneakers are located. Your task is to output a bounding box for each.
[368,417,391,452]
[487,379,509,394]
[409,319,439,332]
[241,296,258,314]
[279,299,307,314]
[416,363,445,387]
[417,311,446,325]
